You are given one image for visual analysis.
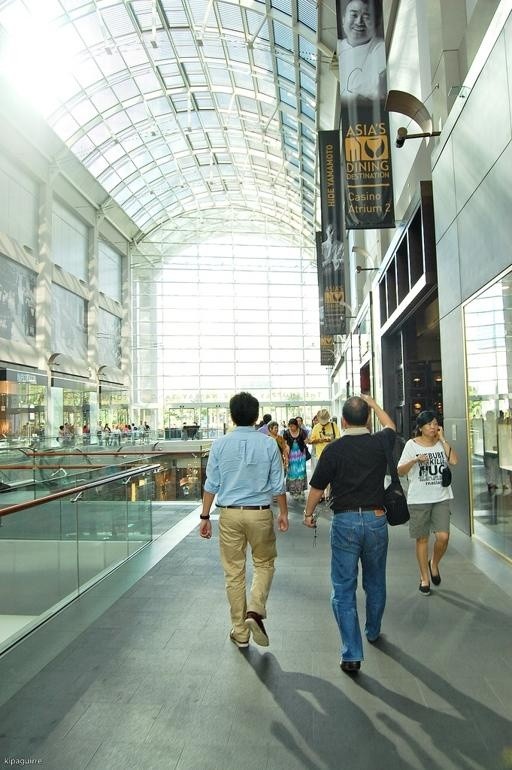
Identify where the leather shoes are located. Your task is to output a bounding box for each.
[340,660,361,672]
[428,559,441,585]
[244,612,269,647]
[230,628,249,648]
[418,581,430,595]
[366,636,379,643]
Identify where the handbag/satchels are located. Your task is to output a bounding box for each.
[305,446,312,461]
[384,482,410,526]
[442,467,451,487]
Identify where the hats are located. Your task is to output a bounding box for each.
[317,409,331,426]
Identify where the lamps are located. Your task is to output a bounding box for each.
[394,126,441,148]
[340,314,356,320]
[333,341,346,344]
[356,264,382,275]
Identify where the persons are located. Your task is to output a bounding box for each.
[200,392,289,648]
[337,1,386,100]
[471,407,512,496]
[257,409,341,503]
[321,224,344,272]
[396,412,457,596]
[303,393,396,670]
[59,422,149,446]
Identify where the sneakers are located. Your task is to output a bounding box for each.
[272,493,332,504]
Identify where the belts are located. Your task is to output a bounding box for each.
[333,504,386,514]
[221,504,270,510]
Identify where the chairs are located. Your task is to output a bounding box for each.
[27,427,152,449]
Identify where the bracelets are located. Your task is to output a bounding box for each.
[441,440,448,443]
[304,510,312,517]
[199,513,209,519]
